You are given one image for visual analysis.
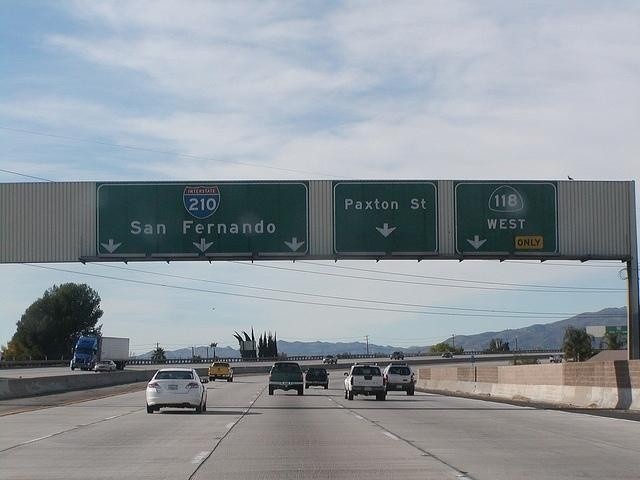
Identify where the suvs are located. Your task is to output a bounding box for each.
[305,367,330,389]
[323,354,338,364]
[382,363,417,396]
[266,362,309,395]
[344,362,385,400]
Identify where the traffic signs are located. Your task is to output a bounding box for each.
[95,180,311,259]
[331,180,439,259]
[453,180,560,259]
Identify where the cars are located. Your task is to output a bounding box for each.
[442,352,454,358]
[95,360,116,373]
[146,367,208,413]
[389,351,404,360]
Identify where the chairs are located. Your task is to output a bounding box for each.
[370,370,376,374]
[163,375,169,378]
[356,370,363,374]
[184,375,191,379]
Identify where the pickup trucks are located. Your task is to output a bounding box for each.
[208,361,236,382]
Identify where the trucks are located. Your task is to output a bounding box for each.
[70,335,129,371]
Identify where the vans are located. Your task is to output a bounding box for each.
[549,355,562,363]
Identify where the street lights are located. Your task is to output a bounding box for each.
[515,334,518,350]
